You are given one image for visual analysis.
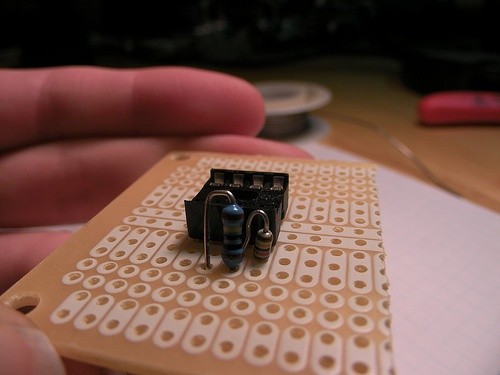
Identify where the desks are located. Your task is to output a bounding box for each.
[282,77,499,214]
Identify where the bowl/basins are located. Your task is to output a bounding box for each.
[255,81,332,137]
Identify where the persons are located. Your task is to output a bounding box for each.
[0,66,315,375]
